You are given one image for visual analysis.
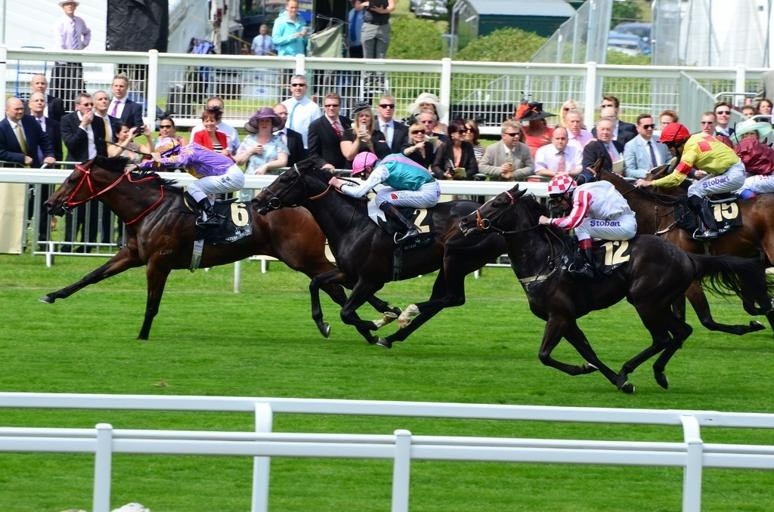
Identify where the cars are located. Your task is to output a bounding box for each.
[579,11,654,65]
[405,1,455,23]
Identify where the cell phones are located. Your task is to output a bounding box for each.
[360,125,367,132]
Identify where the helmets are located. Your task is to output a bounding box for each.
[154,137,181,157]
[352,151,378,176]
[658,123,690,144]
[547,173,577,198]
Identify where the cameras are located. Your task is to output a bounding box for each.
[136,127,144,134]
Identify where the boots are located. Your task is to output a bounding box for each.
[571,238,594,279]
[688,195,719,238]
[196,196,219,226]
[379,200,420,242]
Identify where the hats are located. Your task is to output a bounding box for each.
[415,92,439,106]
[244,108,284,133]
[729,120,772,141]
[59,0,79,7]
[353,101,369,113]
[516,100,556,122]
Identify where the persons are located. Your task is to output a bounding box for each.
[1,70,774,255]
[52,1,91,70]
[250,0,395,102]
[536,174,638,272]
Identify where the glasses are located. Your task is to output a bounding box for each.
[380,104,394,109]
[504,132,520,137]
[717,111,731,115]
[292,84,305,87]
[412,130,426,134]
[160,125,172,129]
[644,124,655,129]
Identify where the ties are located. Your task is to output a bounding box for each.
[333,122,341,137]
[557,152,567,173]
[508,151,515,171]
[104,121,111,146]
[350,11,358,42]
[648,141,657,168]
[16,125,26,156]
[261,37,265,55]
[383,124,389,141]
[288,104,299,130]
[71,20,79,50]
[110,101,120,118]
[279,132,283,144]
[37,119,44,162]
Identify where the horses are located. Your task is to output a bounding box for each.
[249,156,483,345]
[459,156,774,395]
[37,154,404,345]
